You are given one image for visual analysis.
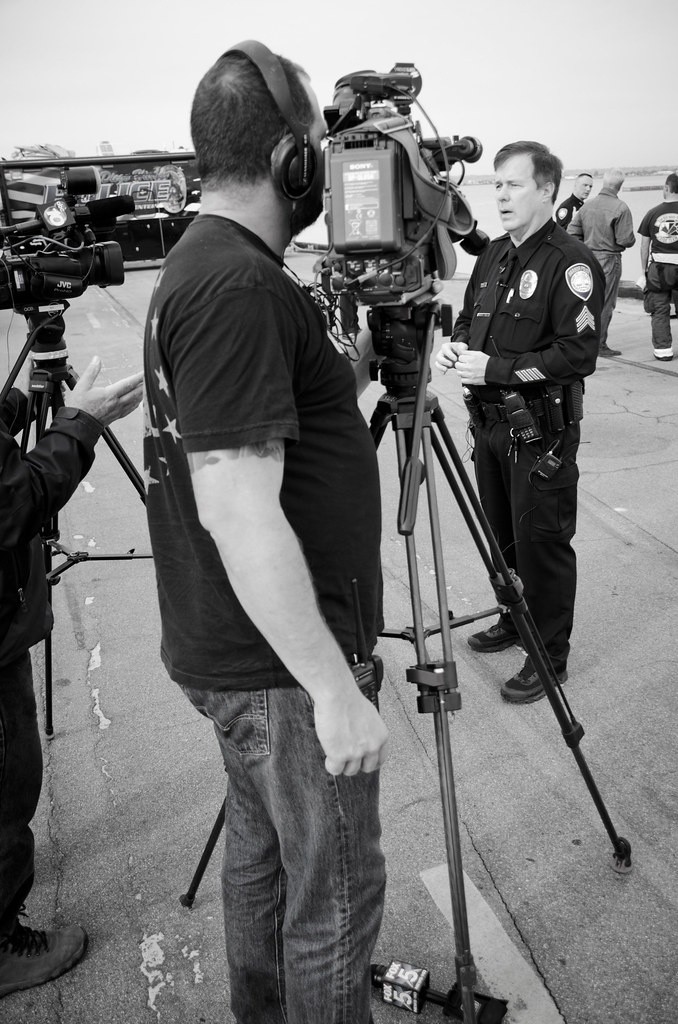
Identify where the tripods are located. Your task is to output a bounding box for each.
[0,300,154,741]
[179,299,633,1024]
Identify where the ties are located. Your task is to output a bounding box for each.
[468,250,517,353]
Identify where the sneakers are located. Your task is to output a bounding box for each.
[467,625,518,653]
[0,907,89,1000]
[501,662,569,703]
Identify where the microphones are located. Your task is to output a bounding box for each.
[72,195,135,219]
[370,960,509,1024]
[457,137,483,163]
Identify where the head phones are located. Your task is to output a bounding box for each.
[222,39,319,201]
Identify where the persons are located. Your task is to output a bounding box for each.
[433,141,606,707]
[637,173,678,362]
[138,37,388,1024]
[555,168,636,358]
[0,356,147,997]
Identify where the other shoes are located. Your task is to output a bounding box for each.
[598,348,622,357]
[653,353,673,361]
